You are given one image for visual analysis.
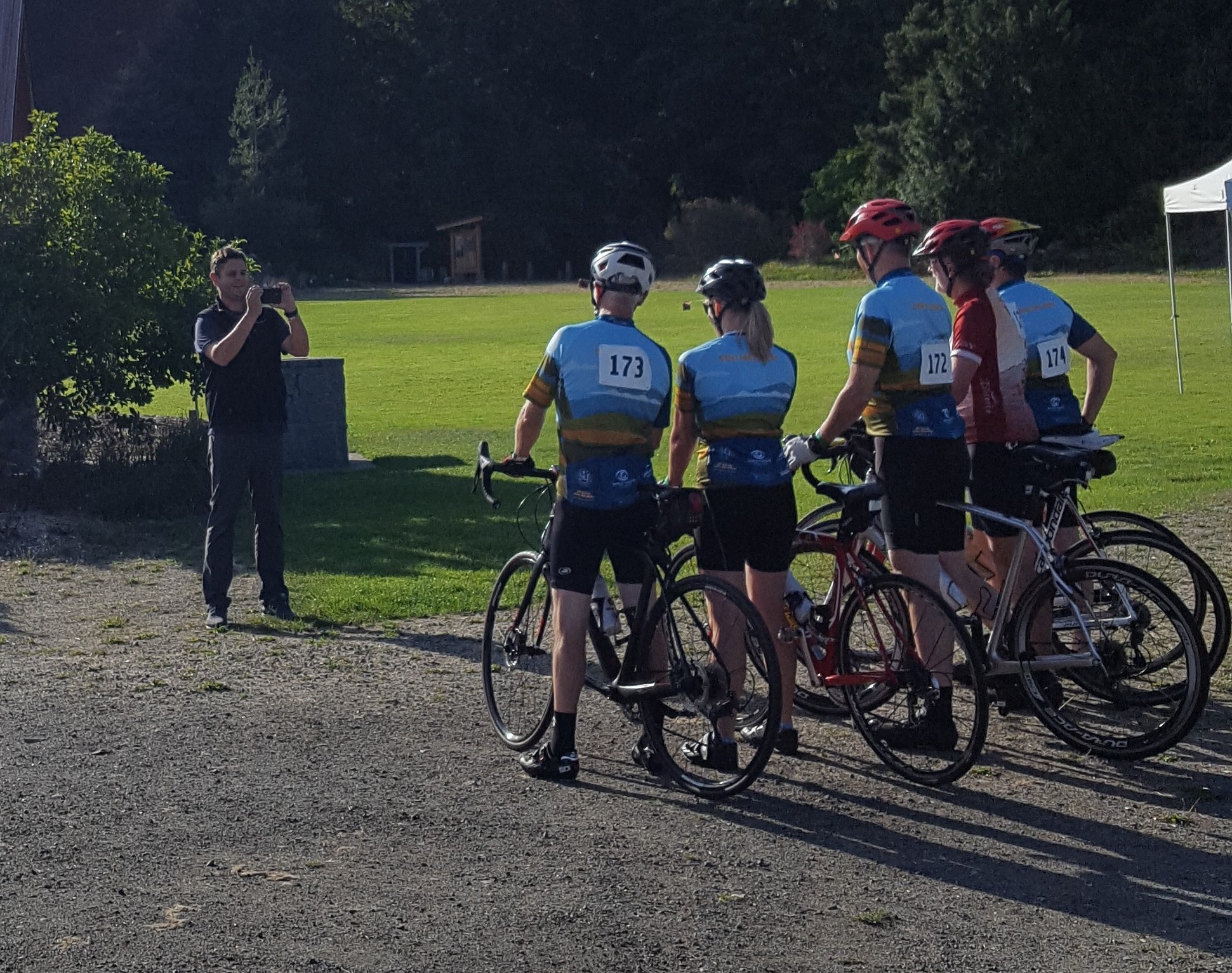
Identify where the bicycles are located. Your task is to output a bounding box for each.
[469,439,782,798]
[744,430,1231,789]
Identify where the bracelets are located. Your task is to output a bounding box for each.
[286,314,300,321]
[284,307,298,318]
[510,452,528,462]
[664,475,670,489]
[816,429,830,447]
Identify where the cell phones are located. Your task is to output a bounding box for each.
[258,289,282,304]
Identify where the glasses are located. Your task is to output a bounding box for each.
[701,302,714,315]
[849,240,863,253]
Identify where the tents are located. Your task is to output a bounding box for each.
[1163,158,1232,395]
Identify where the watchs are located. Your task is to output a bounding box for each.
[807,433,827,455]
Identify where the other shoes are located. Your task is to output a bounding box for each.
[205,597,232,632]
[261,593,303,622]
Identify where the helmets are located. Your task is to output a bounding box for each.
[979,218,1042,259]
[589,241,655,292]
[695,258,767,303]
[839,197,921,241]
[913,220,986,260]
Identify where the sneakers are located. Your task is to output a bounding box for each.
[683,733,738,772]
[740,721,802,755]
[629,738,665,773]
[873,716,960,752]
[520,742,580,779]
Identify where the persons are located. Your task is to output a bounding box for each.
[782,199,1065,752]
[980,218,1118,655]
[194,247,310,629]
[668,257,798,774]
[908,219,1057,668]
[498,242,673,783]
